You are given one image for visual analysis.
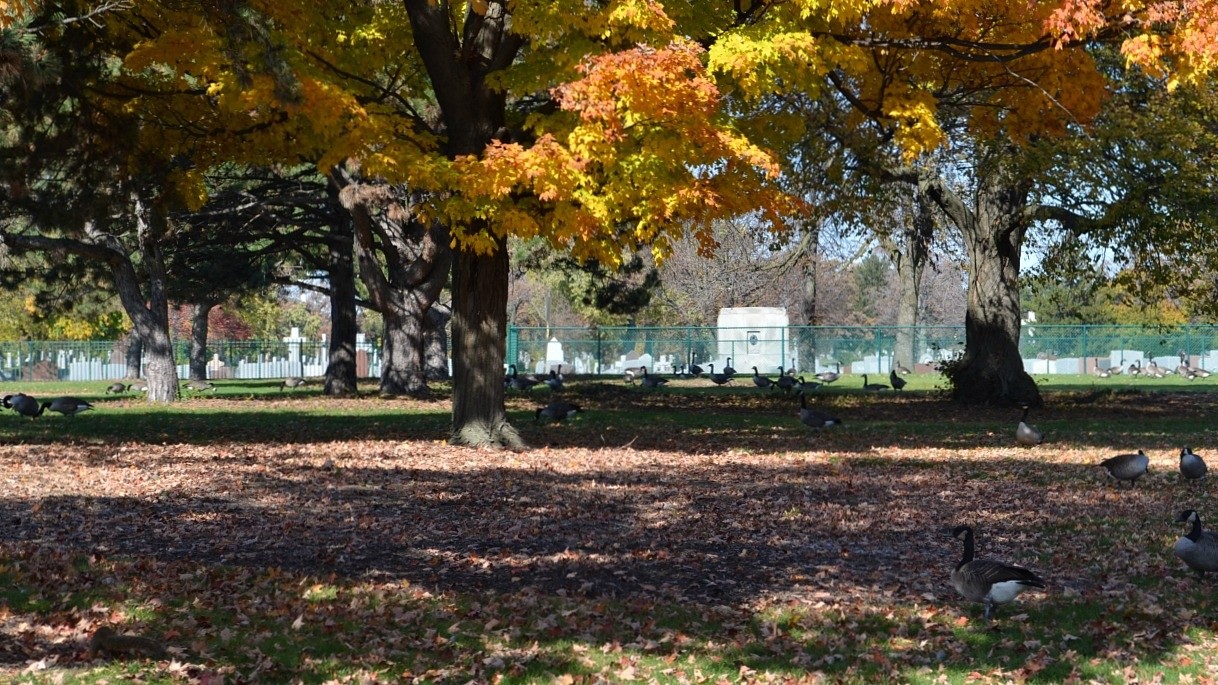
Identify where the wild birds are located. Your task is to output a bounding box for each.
[3,377,308,427]
[947,350,1218,618]
[504,352,912,433]
